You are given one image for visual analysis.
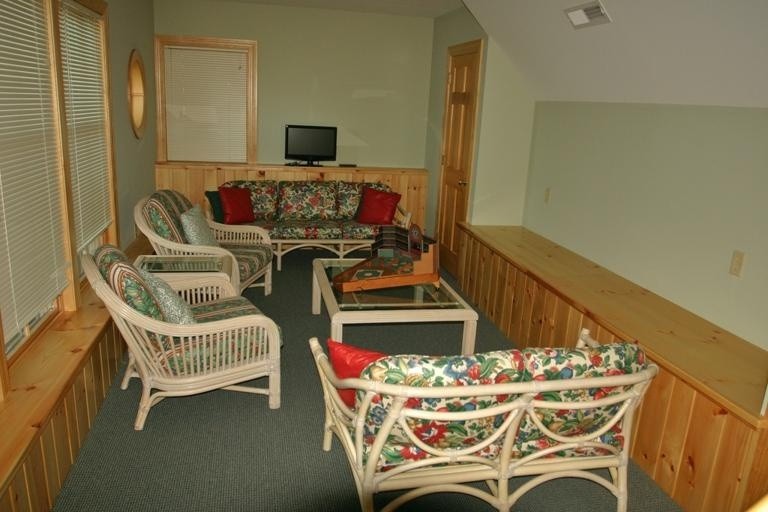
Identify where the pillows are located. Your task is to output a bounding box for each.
[219,186,255,224]
[357,186,401,225]
[138,266,197,325]
[180,202,220,247]
[326,337,385,407]
[204,190,225,223]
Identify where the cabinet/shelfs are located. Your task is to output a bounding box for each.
[455,222,768,512]
[156,161,429,232]
[0,287,124,511]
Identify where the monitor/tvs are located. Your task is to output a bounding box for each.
[284,125,338,167]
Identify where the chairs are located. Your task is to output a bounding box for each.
[82,244,283,432]
[133,189,274,299]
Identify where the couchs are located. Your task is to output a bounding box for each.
[308,336,657,510]
[205,180,412,271]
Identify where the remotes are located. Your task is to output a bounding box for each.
[339,164,357,167]
